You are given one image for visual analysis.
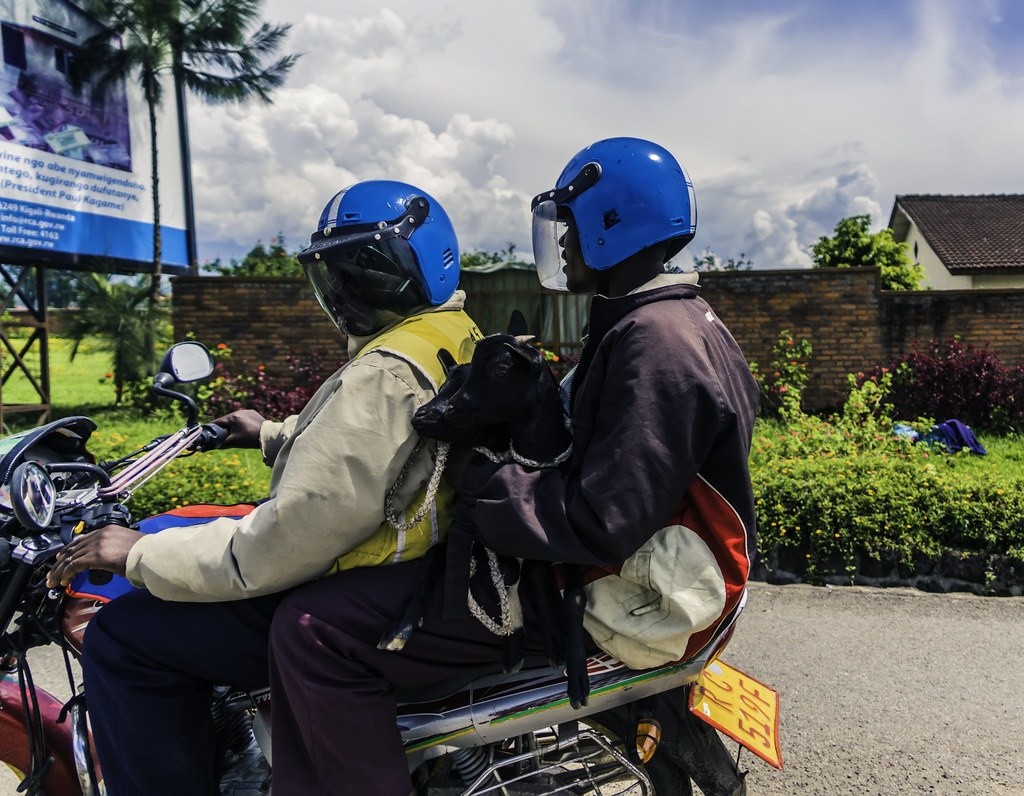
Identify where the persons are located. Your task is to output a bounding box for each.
[270,137,762,796]
[44,181,489,796]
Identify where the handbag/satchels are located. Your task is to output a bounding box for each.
[560,474,748,671]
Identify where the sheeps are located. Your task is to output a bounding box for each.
[413,308,589,711]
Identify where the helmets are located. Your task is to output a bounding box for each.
[536,141,697,273]
[296,180,461,337]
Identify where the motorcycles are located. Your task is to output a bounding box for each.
[0,342,748,796]
